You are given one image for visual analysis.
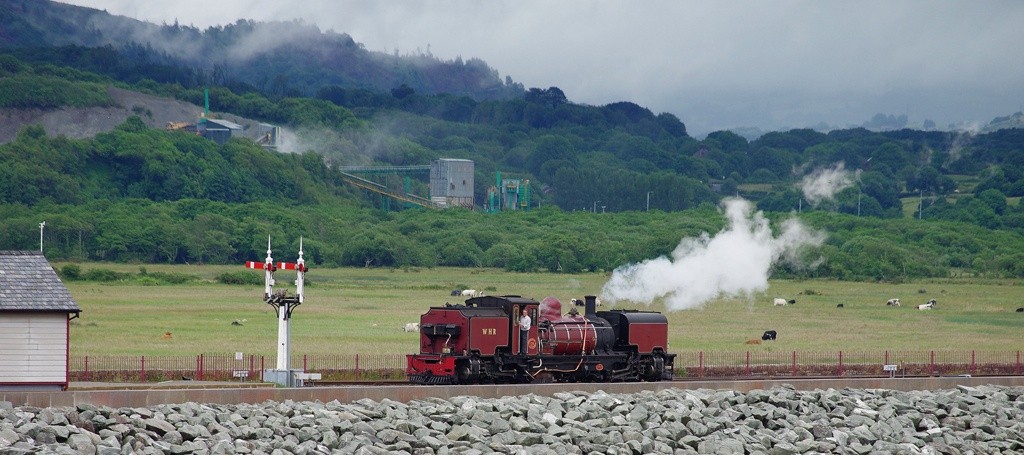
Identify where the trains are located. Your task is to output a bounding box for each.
[402,290,675,381]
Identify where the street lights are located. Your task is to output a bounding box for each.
[39,221,47,253]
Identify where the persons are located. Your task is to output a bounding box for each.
[517,308,531,355]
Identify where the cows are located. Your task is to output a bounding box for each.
[1016,308,1023,312]
[232,321,243,326]
[402,323,419,332]
[886,299,900,307]
[837,304,845,308]
[463,290,477,297]
[774,298,788,307]
[918,299,937,311]
[451,289,462,297]
[762,330,777,340]
[788,300,796,304]
